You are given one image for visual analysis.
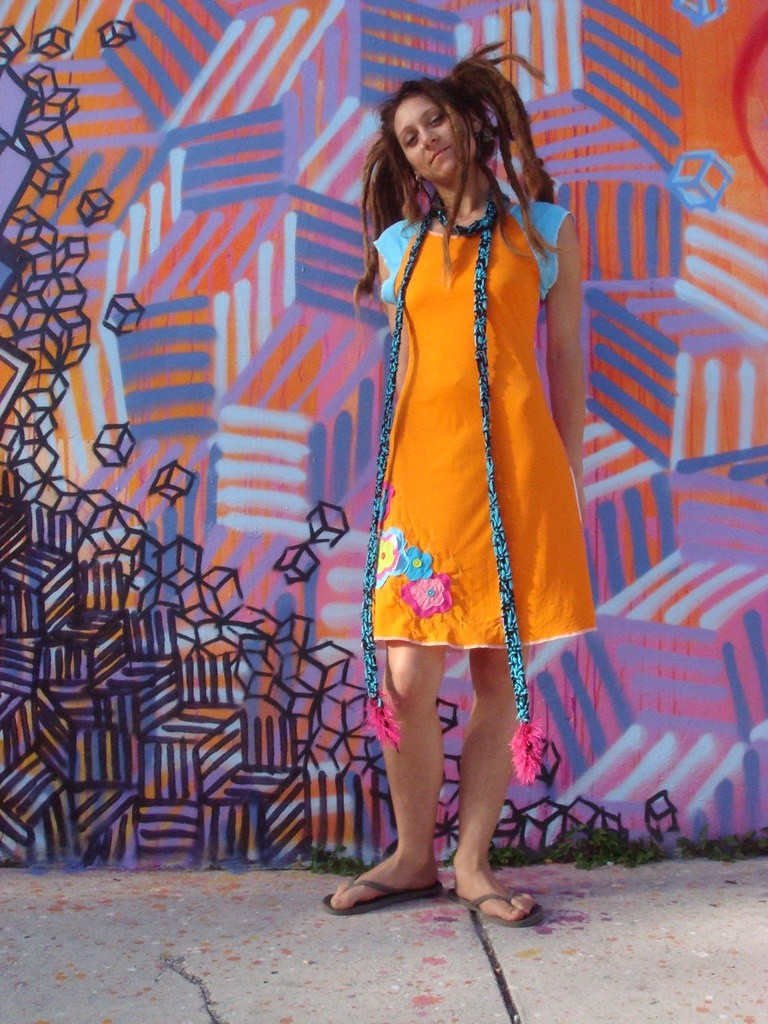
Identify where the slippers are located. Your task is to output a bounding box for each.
[321,873,443,915]
[446,889,543,928]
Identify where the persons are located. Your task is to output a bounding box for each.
[321,42,587,931]
